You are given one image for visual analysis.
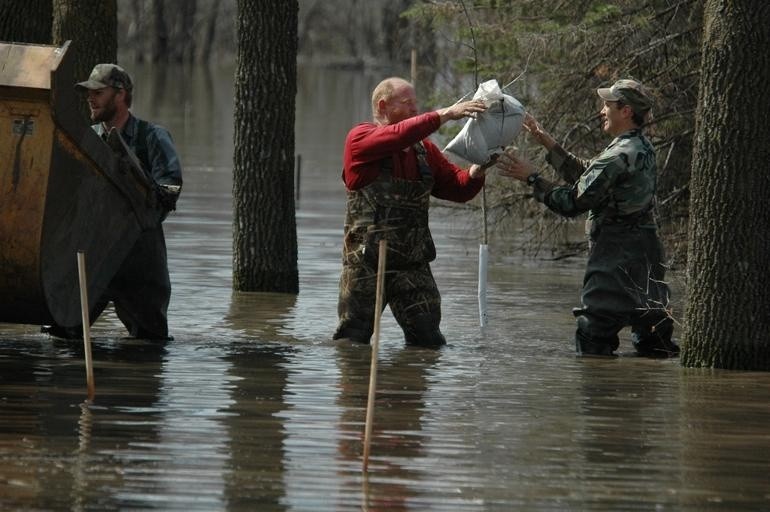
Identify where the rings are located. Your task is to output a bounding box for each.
[508,166,513,172]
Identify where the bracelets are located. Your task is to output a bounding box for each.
[526,172,538,186]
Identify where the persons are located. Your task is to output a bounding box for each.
[331,76,499,346]
[334,342,439,512]
[75,62,182,339]
[574,353,672,510]
[38,335,165,510]
[495,77,683,360]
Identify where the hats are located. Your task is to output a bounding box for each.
[74,62,133,92]
[597,79,653,116]
